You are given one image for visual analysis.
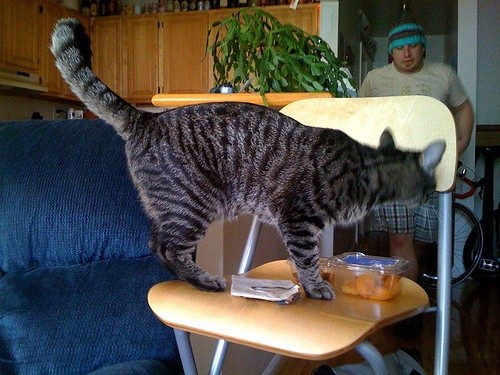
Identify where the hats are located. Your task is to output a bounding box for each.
[388,10,427,55]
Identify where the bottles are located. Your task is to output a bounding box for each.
[219,81,233,94]
[56,0,320,18]
[210,85,220,94]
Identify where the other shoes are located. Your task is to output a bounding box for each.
[392,316,424,339]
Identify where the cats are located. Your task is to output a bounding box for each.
[50,17,447,300]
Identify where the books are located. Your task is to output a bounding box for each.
[231,275,300,301]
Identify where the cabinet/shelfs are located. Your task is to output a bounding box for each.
[0,0,320,104]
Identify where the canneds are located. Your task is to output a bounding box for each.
[198,2,203,10]
[152,3,157,13]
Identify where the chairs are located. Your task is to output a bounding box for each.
[147,95,457,375]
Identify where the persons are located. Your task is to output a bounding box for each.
[90,0,210,16]
[356,16,475,369]
[251,284,299,298]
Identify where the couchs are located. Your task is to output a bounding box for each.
[0,119,197,375]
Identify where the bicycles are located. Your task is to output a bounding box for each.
[353,160,485,290]
[463,203,500,290]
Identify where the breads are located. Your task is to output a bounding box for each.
[342,271,402,300]
